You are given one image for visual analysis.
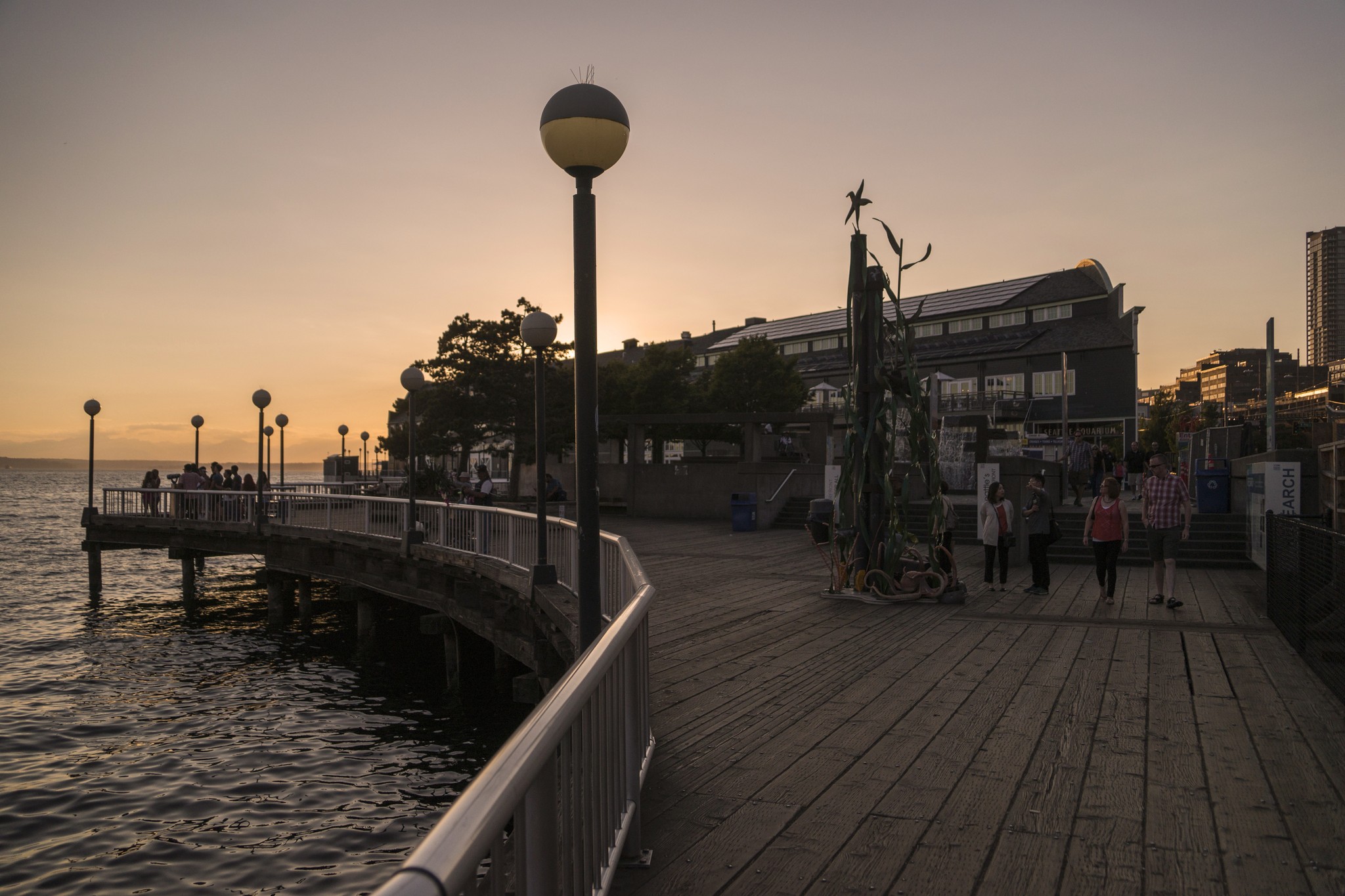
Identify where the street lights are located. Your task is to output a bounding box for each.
[359,448,362,470]
[191,414,204,468]
[348,450,351,456]
[360,430,370,503]
[538,81,632,654]
[252,389,271,524]
[344,449,346,456]
[374,449,380,485]
[384,452,386,461]
[338,424,349,502]
[366,450,369,476]
[274,413,290,500]
[263,425,274,481]
[400,366,425,544]
[520,312,557,586]
[80,398,101,524]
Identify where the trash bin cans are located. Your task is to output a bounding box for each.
[731,492,758,532]
[808,499,833,545]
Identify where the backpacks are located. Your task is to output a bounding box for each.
[941,496,960,530]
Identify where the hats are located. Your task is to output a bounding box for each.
[474,465,487,474]
[459,472,470,479]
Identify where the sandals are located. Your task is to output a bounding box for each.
[1149,594,1165,604]
[1167,597,1183,609]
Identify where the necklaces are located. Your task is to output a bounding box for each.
[1103,496,1115,504]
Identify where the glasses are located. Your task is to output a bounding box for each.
[1075,434,1082,437]
[1149,463,1165,468]
[1101,483,1109,487]
[1152,444,1158,446]
[1029,481,1039,484]
[1092,448,1098,450]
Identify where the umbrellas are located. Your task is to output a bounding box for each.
[920,371,957,395]
[809,382,838,403]
[842,381,854,388]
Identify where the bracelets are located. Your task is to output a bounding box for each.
[1090,466,1094,469]
[1083,535,1089,538]
[1124,539,1129,543]
[1184,524,1190,528]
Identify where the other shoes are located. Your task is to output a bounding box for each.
[1078,500,1084,506]
[1023,585,1037,592]
[999,588,1006,591]
[1132,495,1136,500]
[1136,494,1143,501]
[1106,596,1114,605]
[1099,585,1106,598]
[988,588,996,591]
[1032,585,1049,594]
[1074,496,1082,505]
[1092,498,1094,501]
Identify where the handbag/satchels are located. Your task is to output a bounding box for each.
[1090,495,1100,520]
[1047,519,1064,546]
[1002,532,1017,548]
[556,490,567,501]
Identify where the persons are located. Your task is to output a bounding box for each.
[1142,453,1192,609]
[1021,472,1051,595]
[781,431,795,455]
[930,481,954,578]
[1089,441,1161,501]
[980,481,1014,592]
[373,478,387,497]
[764,423,774,435]
[545,473,563,502]
[445,464,494,555]
[1053,430,1094,507]
[1083,477,1130,605]
[139,461,271,525]
